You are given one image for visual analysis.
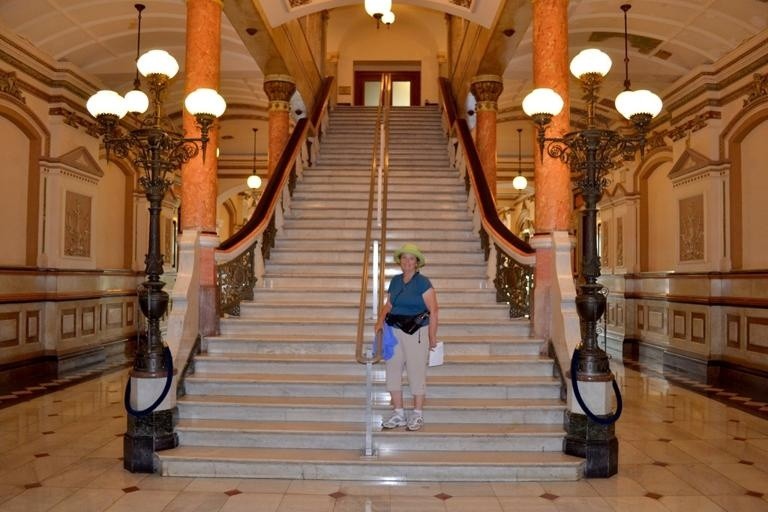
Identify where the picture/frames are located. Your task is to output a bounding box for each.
[61,190,95,260]
[596,207,629,276]
[676,191,707,266]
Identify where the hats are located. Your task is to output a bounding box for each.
[394,243,426,268]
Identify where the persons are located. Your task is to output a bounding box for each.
[372,242,439,430]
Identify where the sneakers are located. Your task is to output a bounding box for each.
[406,414,426,431]
[382,413,408,429]
